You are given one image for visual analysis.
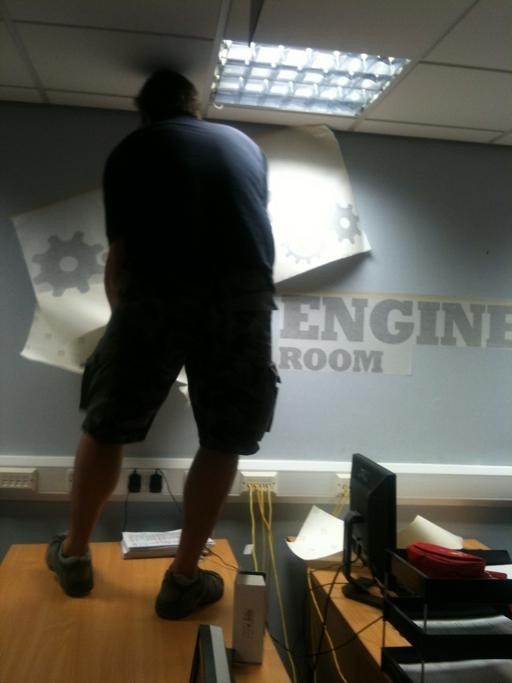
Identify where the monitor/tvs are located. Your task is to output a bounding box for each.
[189,624,236,683]
[343,453,397,610]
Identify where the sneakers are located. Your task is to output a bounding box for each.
[42,530,95,598]
[154,565,225,620]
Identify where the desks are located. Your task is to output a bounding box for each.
[0,538,293,683]
[286,535,491,683]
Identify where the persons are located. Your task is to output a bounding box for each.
[42,66,283,621]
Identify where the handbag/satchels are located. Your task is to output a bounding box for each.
[405,541,509,582]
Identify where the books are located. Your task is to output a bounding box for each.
[119,528,214,560]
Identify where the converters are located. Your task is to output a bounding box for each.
[149,474,161,493]
[129,474,141,492]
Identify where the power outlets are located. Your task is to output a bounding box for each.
[126,469,166,494]
